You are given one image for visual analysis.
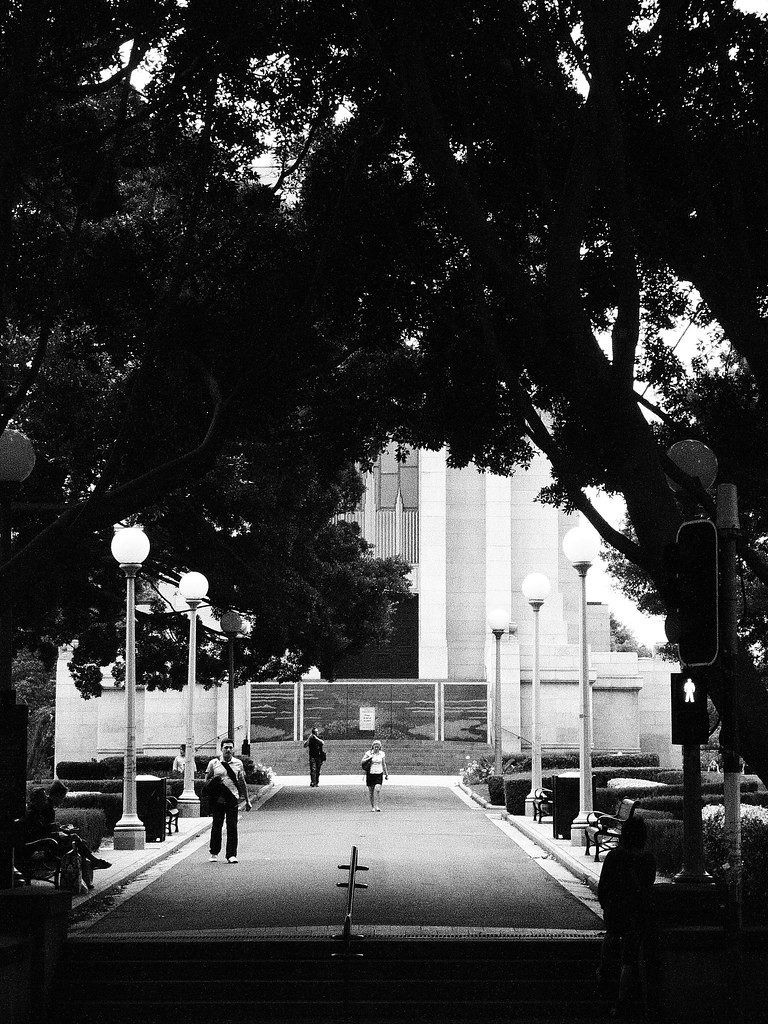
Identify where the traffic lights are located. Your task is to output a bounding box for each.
[670,672,710,745]
[674,520,720,666]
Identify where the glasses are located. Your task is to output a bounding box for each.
[180,748,183,750]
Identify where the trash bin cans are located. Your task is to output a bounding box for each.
[552,772,596,839]
[122,774,167,842]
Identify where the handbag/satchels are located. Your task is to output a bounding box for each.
[362,758,372,771]
[235,781,246,797]
[319,752,326,761]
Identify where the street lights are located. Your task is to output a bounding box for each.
[219,611,243,740]
[110,527,151,829]
[560,526,602,825]
[178,571,210,800]
[485,608,511,780]
[0,428,37,890]
[519,571,552,799]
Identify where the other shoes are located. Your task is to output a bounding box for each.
[90,859,111,870]
[310,782,318,787]
[376,806,381,812]
[372,806,376,812]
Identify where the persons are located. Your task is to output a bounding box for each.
[172,744,197,773]
[26,788,113,889]
[708,760,719,772]
[597,817,656,1003]
[242,739,250,757]
[360,740,388,811]
[205,738,252,863]
[303,727,325,787]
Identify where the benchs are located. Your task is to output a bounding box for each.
[584,796,641,862]
[166,796,180,834]
[533,788,552,823]
[11,818,62,889]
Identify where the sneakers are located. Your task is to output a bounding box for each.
[228,856,238,863]
[209,853,218,862]
